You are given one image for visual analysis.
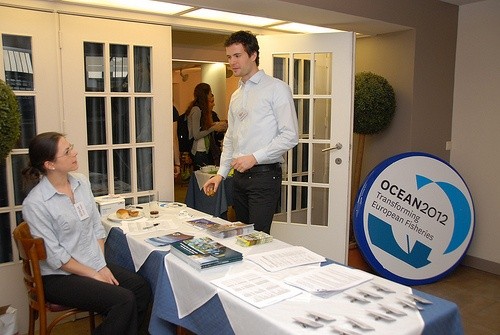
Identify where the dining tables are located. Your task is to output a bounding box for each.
[104,199,464,335]
[185,170,233,216]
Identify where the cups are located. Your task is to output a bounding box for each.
[220,120,227,132]
[149,189,160,219]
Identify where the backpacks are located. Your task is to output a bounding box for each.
[177,105,204,152]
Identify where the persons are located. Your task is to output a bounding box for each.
[203,31,300,235]
[173,83,228,178]
[21,131,152,335]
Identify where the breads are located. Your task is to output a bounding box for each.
[117,208,129,219]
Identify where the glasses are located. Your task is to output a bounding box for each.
[55,144,74,160]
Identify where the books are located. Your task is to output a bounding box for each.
[143,231,243,269]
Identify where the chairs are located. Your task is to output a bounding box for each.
[13,222,95,335]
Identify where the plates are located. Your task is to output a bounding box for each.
[107,213,144,221]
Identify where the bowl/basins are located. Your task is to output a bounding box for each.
[200,165,215,172]
